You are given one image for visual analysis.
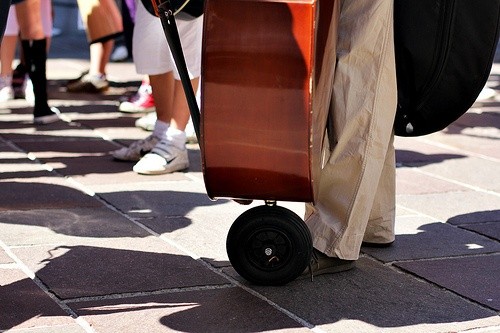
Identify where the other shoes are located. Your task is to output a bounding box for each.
[67,73,109,93]
[33,107,61,124]
[477,87,495,100]
[134,112,157,130]
[363,242,393,248]
[299,249,355,282]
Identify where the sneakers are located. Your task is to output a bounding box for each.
[118,85,155,112]
[113,136,160,161]
[133,143,189,175]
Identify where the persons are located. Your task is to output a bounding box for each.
[0,0,205,175]
[292,0,398,282]
